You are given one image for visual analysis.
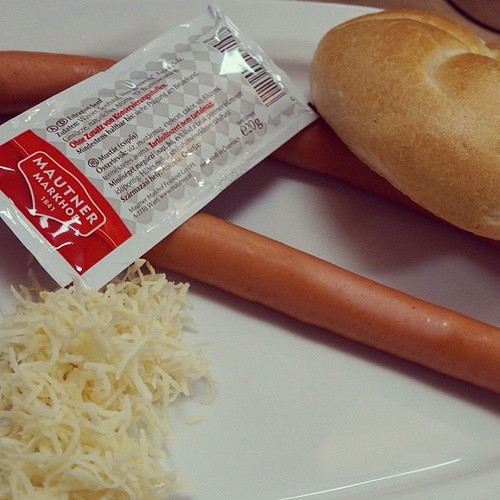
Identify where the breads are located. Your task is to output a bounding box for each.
[308,8,499,243]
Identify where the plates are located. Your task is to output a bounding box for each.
[2,1,500,500]
